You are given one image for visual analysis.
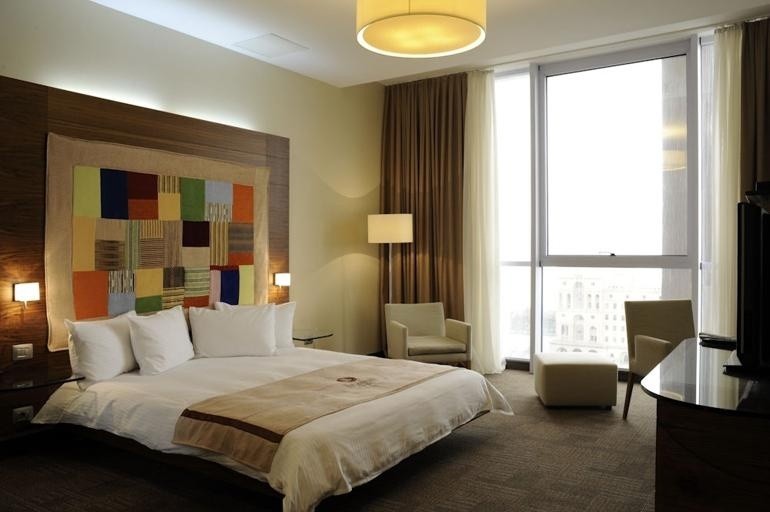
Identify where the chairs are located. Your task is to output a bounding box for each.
[623,299,696,421]
[385,302,471,369]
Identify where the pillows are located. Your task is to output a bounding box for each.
[214,301,298,348]
[124,304,195,376]
[189,302,282,357]
[63,310,138,392]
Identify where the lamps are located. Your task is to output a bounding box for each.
[14,282,40,308]
[275,273,291,290]
[356,0,487,58]
[368,214,413,304]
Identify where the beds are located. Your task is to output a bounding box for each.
[30,344,514,512]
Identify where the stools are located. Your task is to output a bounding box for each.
[535,351,617,411]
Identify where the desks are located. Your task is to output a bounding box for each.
[640,336,770,512]
